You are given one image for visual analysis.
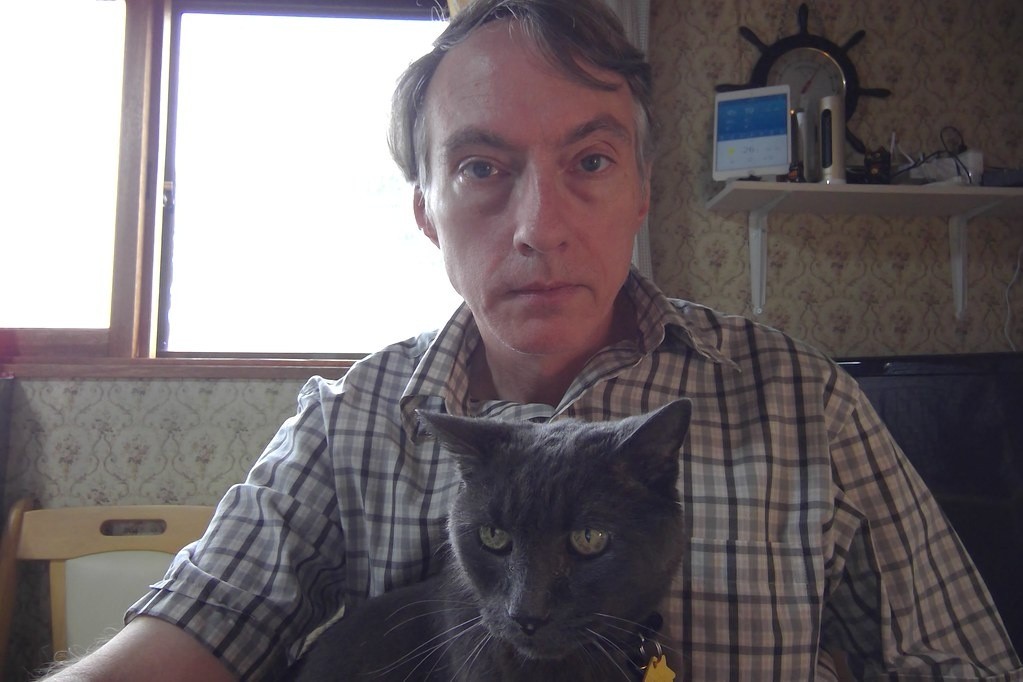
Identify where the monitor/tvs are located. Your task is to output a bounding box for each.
[830,352,1023,666]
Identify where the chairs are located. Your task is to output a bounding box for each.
[0,496,217,682]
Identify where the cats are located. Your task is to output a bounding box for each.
[277,398,692,682]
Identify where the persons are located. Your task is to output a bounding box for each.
[38,0,1023,682]
